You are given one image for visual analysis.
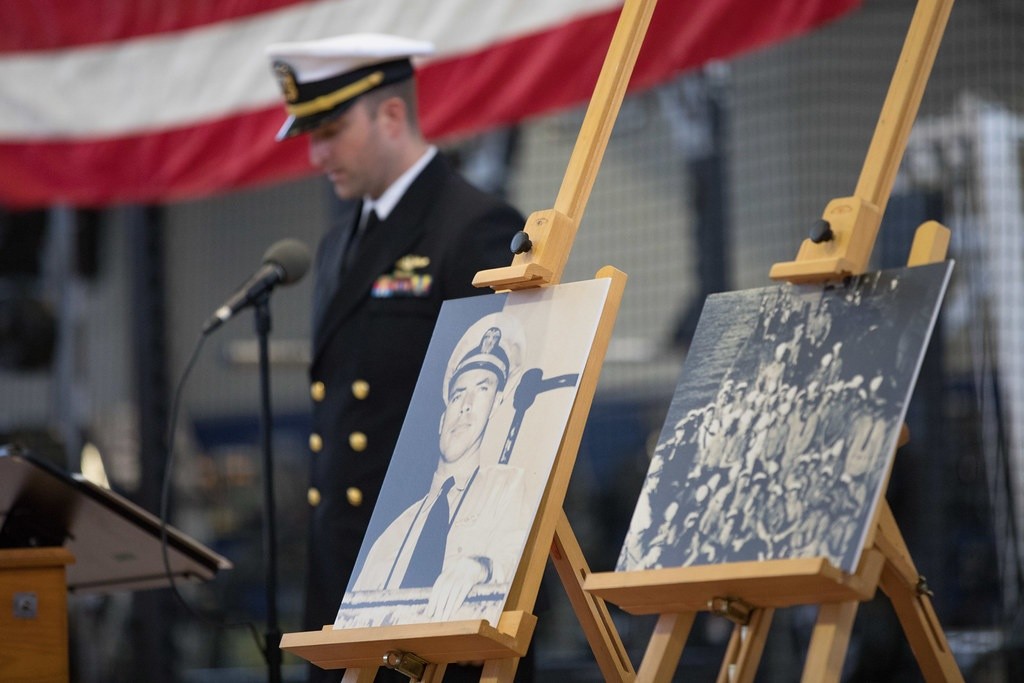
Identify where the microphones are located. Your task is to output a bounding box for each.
[203,238,309,335]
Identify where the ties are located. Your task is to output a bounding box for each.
[399,476,454,589]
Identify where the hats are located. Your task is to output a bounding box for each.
[443,311,526,406]
[263,32,437,142]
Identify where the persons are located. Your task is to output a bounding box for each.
[352,310,537,622]
[617,288,891,569]
[267,34,525,683]
